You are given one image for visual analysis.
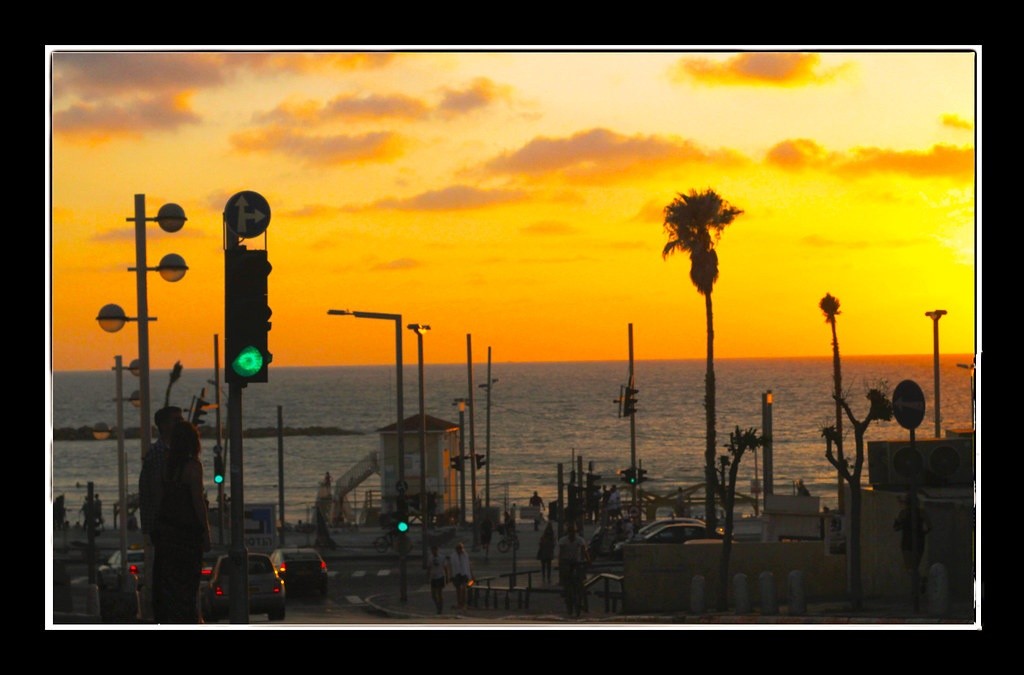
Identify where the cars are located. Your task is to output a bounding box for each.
[266,547,329,592]
[93,549,216,624]
[627,515,738,545]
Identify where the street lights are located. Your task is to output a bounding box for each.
[407,324,433,572]
[94,194,190,457]
[326,309,408,602]
[956,363,976,486]
[454,397,470,523]
[925,309,948,438]
[94,355,141,574]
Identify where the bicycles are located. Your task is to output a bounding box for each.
[373,526,413,555]
[497,525,520,554]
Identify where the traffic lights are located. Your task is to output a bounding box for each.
[192,398,211,424]
[628,469,638,485]
[623,386,640,418]
[213,456,225,484]
[637,469,648,484]
[395,493,409,536]
[620,467,632,484]
[476,454,486,470]
[224,245,274,383]
[450,455,463,472]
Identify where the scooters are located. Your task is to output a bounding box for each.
[584,523,624,563]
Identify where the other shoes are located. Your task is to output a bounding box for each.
[921,577,929,593]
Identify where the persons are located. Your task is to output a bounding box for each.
[480,512,495,544]
[796,478,810,496]
[823,505,829,512]
[511,503,516,518]
[425,546,449,584]
[223,494,231,505]
[205,494,209,511]
[450,542,471,610]
[337,512,345,522]
[79,494,104,532]
[557,519,593,599]
[592,484,624,528]
[428,558,447,615]
[631,503,642,538]
[325,472,334,489]
[429,491,437,523]
[539,523,557,583]
[503,510,518,543]
[529,491,546,531]
[295,520,303,531]
[893,493,931,607]
[676,486,686,516]
[138,405,213,625]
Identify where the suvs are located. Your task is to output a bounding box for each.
[202,552,287,622]
